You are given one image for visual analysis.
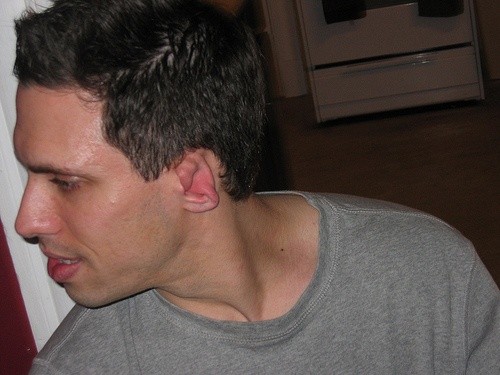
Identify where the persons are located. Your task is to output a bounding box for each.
[13,0,500,375]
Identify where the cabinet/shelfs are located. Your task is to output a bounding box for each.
[295,0,485,124]
